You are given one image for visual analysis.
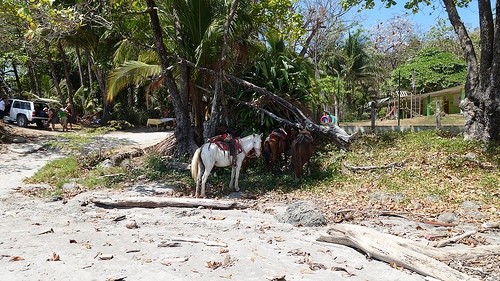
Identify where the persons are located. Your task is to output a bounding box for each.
[0,95,77,133]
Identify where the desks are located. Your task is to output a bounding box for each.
[147,118,176,130]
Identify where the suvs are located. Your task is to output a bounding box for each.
[4,98,62,129]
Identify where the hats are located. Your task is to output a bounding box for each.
[43,106,47,110]
[67,102,71,105]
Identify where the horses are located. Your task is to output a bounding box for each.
[190,132,263,198]
[263,125,314,181]
